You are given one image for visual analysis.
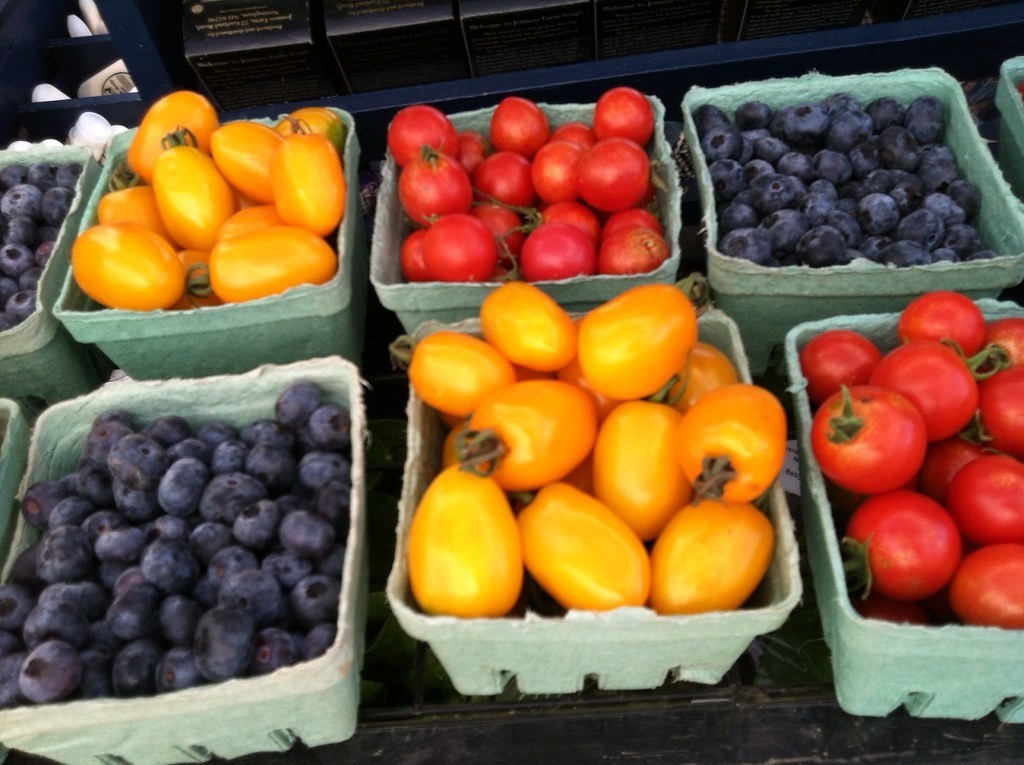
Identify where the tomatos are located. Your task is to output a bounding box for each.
[69,90,349,311]
[800,291,1024,630]
[405,278,786,618]
[388,85,670,282]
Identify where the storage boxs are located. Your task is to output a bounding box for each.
[679,63,1024,370]
[1,145,108,418]
[52,106,364,377]
[786,296,1024,723]
[0,355,366,765]
[368,92,683,342]
[385,306,800,698]
